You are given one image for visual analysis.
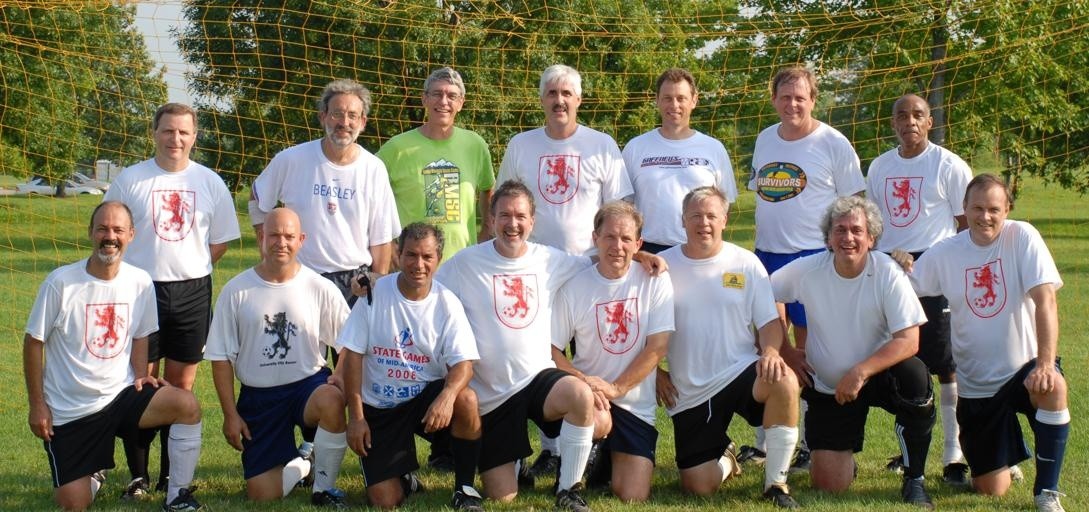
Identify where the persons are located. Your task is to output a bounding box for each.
[621,72,738,255]
[101,103,240,500]
[336,222,485,512]
[21,203,205,511]
[247,79,400,442]
[768,196,937,510]
[656,187,800,511]
[734,67,867,476]
[372,68,496,273]
[891,172,1071,511]
[867,92,975,484]
[352,178,669,512]
[494,65,634,476]
[204,206,352,511]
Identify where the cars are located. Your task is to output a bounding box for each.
[14,176,104,196]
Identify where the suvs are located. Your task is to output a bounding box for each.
[26,168,111,194]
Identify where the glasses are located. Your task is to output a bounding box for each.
[326,109,361,123]
[425,91,460,104]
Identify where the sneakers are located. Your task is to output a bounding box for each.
[723,442,741,476]
[943,462,971,492]
[530,450,559,477]
[451,486,482,512]
[520,456,534,492]
[162,489,213,512]
[1009,465,1023,480]
[1033,491,1064,512]
[790,447,811,471]
[92,470,105,501]
[312,489,354,512]
[298,443,315,486]
[121,478,154,501]
[409,470,425,492]
[886,453,906,474]
[900,481,935,510]
[764,483,804,511]
[557,487,589,512]
[739,446,766,466]
[156,477,198,493]
[587,440,612,496]
